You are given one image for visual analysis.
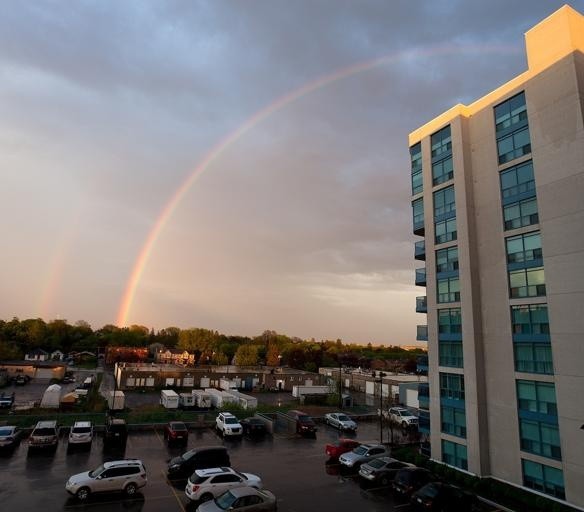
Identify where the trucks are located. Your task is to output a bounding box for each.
[159,379,258,412]
[106,390,125,413]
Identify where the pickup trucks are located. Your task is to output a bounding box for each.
[377,407,420,429]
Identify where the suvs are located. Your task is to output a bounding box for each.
[185,467,263,504]
[215,412,244,440]
[27,420,63,451]
[238,417,267,437]
[67,421,94,451]
[104,419,128,444]
[65,459,148,502]
[166,445,232,483]
[1,391,15,410]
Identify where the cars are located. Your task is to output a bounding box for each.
[163,421,188,444]
[195,487,278,512]
[325,438,470,512]
[0,426,23,452]
[15,374,28,386]
[322,412,358,433]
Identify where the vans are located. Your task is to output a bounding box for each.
[287,410,317,433]
